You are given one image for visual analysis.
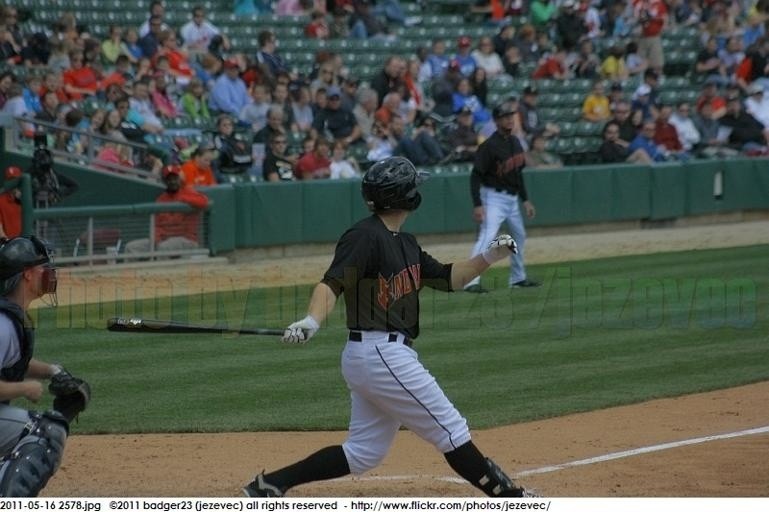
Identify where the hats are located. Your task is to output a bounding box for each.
[325,87,341,100]
[162,164,179,176]
[224,58,239,70]
[3,166,22,181]
[459,36,470,46]
[493,102,518,117]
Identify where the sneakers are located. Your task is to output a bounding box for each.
[509,279,544,290]
[241,468,285,498]
[515,484,543,498]
[463,284,488,295]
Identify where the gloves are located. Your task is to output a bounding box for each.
[281,315,320,345]
[481,234,518,265]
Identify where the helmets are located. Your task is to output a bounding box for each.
[361,156,431,212]
[1,234,59,310]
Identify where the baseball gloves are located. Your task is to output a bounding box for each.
[48,368,92,422]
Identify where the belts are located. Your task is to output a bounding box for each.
[496,188,516,195]
[349,331,410,345]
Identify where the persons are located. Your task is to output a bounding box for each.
[1,2,768,293]
[0,236,91,497]
[240,157,536,497]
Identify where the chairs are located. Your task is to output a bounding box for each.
[1,0,702,174]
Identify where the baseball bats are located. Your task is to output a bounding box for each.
[107,319,308,342]
